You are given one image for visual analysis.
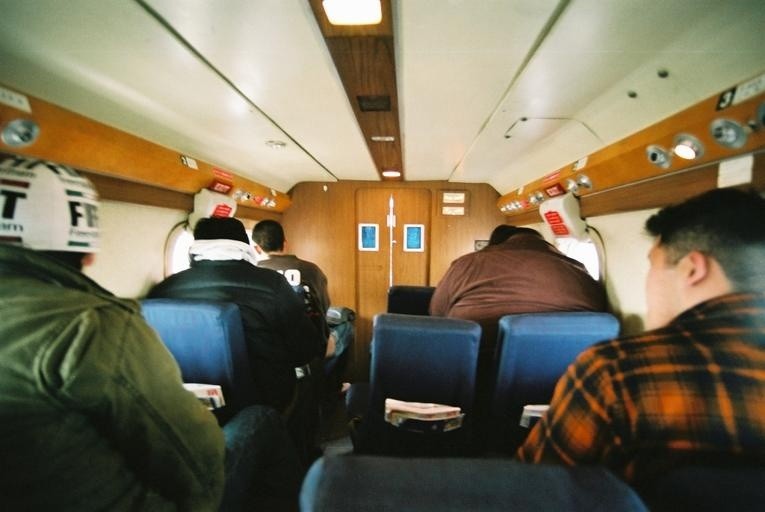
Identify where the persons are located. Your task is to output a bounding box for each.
[517,182,764,511]
[429,225,607,342]
[144,216,322,427]
[0,154,285,512]
[252,219,356,361]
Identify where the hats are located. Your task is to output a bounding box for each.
[0,155,101,255]
